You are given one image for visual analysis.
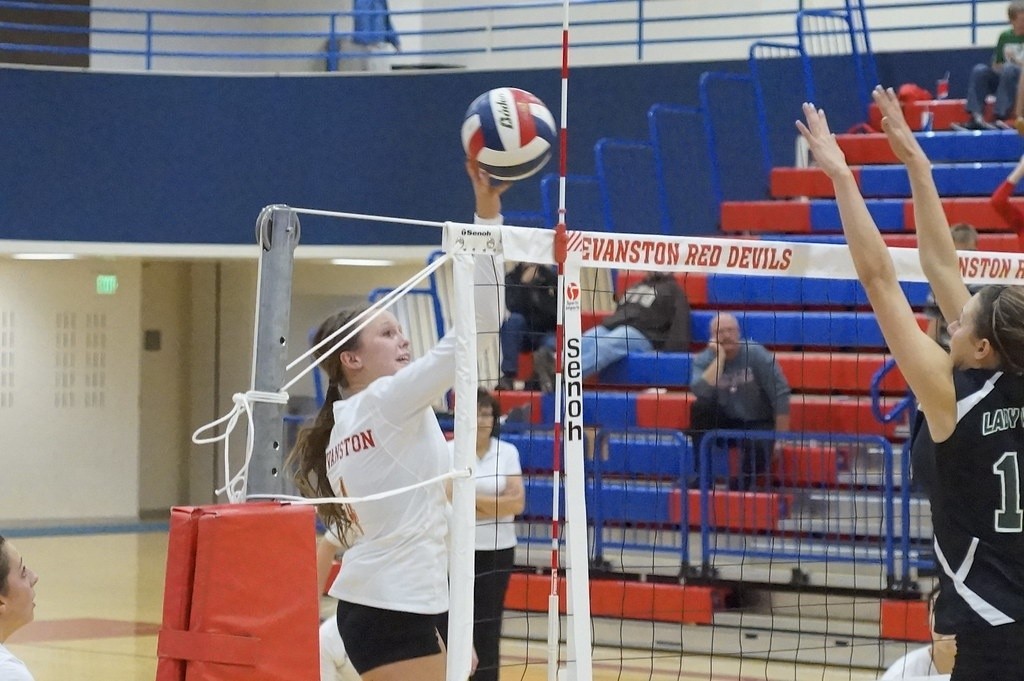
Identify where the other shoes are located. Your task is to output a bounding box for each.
[950,117,986,132]
[494,377,514,391]
[688,473,712,487]
[524,373,544,392]
[533,345,557,395]
[729,478,750,490]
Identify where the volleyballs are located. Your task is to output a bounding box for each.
[460,85,559,181]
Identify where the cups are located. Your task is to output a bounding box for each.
[920,111,933,133]
[937,80,948,100]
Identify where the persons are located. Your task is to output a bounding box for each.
[446,387,525,681]
[991,155,1024,253]
[674,313,790,491]
[926,225,981,355]
[534,269,689,394]
[495,262,566,393]
[951,1,1024,131]
[316,519,478,676]
[283,157,512,681]
[881,582,957,681]
[796,84,1024,681]
[1,536,38,681]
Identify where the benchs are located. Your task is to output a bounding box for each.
[438,97,1024,640]
